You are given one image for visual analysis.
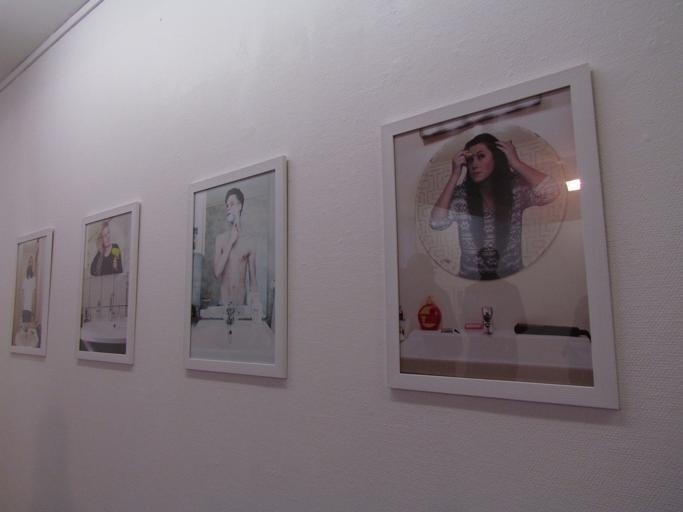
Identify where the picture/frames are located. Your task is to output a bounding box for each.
[183,154,289,379]
[9,227,54,358]
[378,62,623,413]
[75,200,143,367]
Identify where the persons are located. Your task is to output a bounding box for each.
[429,135,559,284]
[214,187,257,312]
[459,246,528,381]
[89,222,123,277]
[561,293,594,386]
[398,252,460,376]
[17,255,36,321]
